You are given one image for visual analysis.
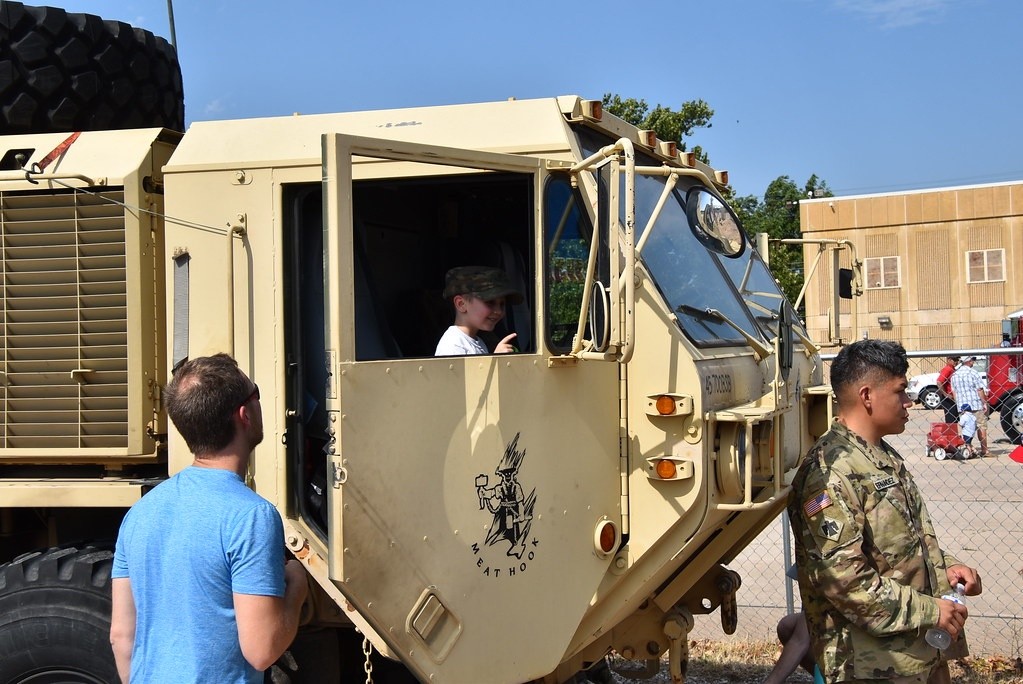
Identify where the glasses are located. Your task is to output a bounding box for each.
[953,359,959,362]
[238,383,262,408]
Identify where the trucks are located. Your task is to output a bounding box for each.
[0,1,864,684]
[985,311,1023,447]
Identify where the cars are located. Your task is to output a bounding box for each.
[904,354,987,410]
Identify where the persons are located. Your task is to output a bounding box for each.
[958,403,977,458]
[937,355,996,457]
[434,266,525,357]
[109,355,308,684]
[762,340,982,684]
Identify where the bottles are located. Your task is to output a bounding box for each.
[925,583,965,651]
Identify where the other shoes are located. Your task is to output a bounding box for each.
[980,451,995,457]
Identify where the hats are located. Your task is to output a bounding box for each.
[442,266,523,306]
[948,355,961,359]
[786,562,798,580]
[960,356,976,362]
[961,404,972,411]
